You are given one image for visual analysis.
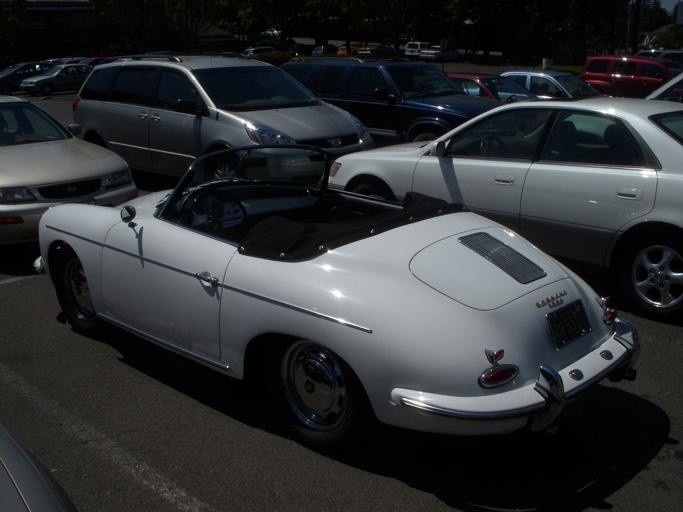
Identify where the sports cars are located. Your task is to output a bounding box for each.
[36,144,641,442]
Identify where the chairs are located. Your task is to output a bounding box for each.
[549,120,577,160]
[604,124,639,166]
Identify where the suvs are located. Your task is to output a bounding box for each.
[72,50,373,180]
[265,55,518,151]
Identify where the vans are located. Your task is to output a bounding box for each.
[582,53,670,98]
[405,41,429,58]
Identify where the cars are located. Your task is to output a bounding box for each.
[446,69,538,103]
[420,46,441,59]
[324,96,683,322]
[1,57,92,128]
[312,42,382,58]
[499,69,602,100]
[1,96,138,249]
